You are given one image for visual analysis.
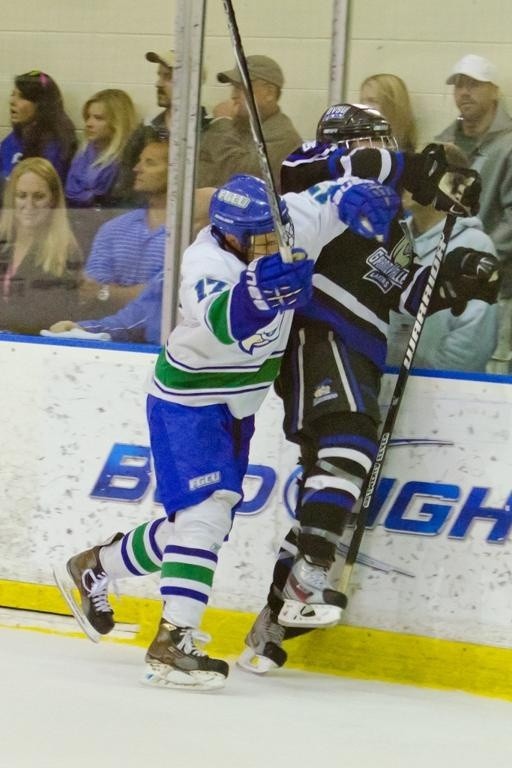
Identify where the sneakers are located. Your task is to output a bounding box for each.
[147,622,227,676]
[244,608,287,667]
[281,559,346,607]
[66,546,114,634]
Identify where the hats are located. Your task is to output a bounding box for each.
[218,55,283,88]
[445,54,496,85]
[146,50,205,84]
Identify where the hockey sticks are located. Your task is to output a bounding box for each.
[268,203,462,629]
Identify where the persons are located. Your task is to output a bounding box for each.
[50,187,219,346]
[243,101,504,669]
[385,141,501,373]
[2,71,76,211]
[64,89,140,209]
[436,54,511,301]
[109,51,213,209]
[200,55,303,196]
[362,73,420,158]
[2,157,84,336]
[82,128,167,318]
[63,174,402,683]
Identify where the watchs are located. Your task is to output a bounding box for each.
[96,284,109,305]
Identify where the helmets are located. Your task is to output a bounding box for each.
[317,104,398,152]
[208,175,295,253]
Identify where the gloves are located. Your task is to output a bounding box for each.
[404,144,481,218]
[422,248,500,315]
[242,249,312,317]
[338,183,400,243]
